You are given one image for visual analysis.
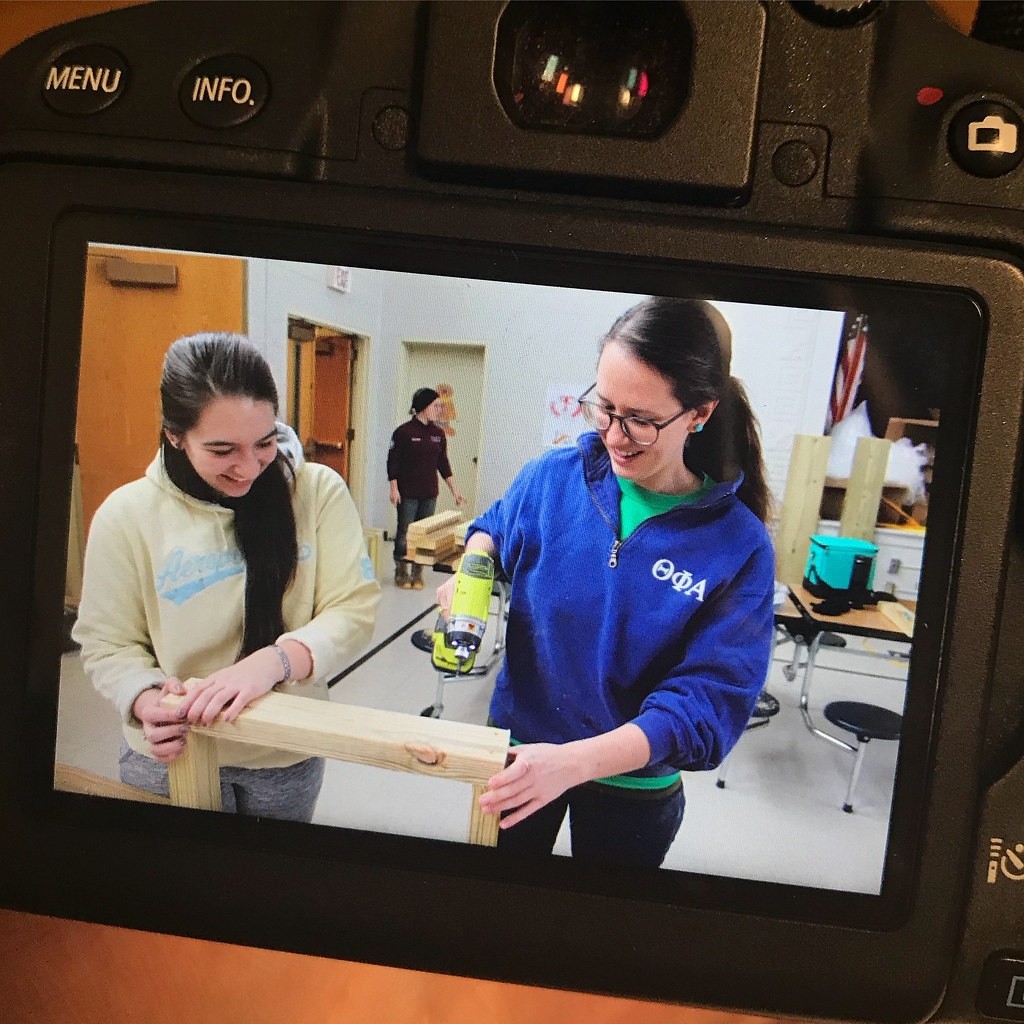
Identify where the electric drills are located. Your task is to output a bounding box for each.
[430,546,496,680]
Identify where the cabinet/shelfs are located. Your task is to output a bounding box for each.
[826,521,924,592]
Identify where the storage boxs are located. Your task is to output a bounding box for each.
[805,535,877,595]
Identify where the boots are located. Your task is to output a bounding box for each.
[393,559,411,588]
[410,562,424,590]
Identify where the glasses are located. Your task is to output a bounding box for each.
[578,382,692,445]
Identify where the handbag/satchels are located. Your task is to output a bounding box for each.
[802,534,880,601]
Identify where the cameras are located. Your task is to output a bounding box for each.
[0,0,1022,1024]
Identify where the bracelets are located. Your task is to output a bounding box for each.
[272,643,291,683]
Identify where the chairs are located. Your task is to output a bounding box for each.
[788,586,901,812]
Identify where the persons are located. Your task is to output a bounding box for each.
[69,330,383,821]
[436,295,775,868]
[386,387,463,590]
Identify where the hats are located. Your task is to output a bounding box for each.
[407,389,438,414]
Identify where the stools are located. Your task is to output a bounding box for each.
[412,629,499,720]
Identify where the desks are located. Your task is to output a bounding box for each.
[772,585,917,642]
[787,585,918,642]
[395,550,801,620]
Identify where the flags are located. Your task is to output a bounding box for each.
[831,314,869,426]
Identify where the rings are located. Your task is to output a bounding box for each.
[524,760,529,770]
